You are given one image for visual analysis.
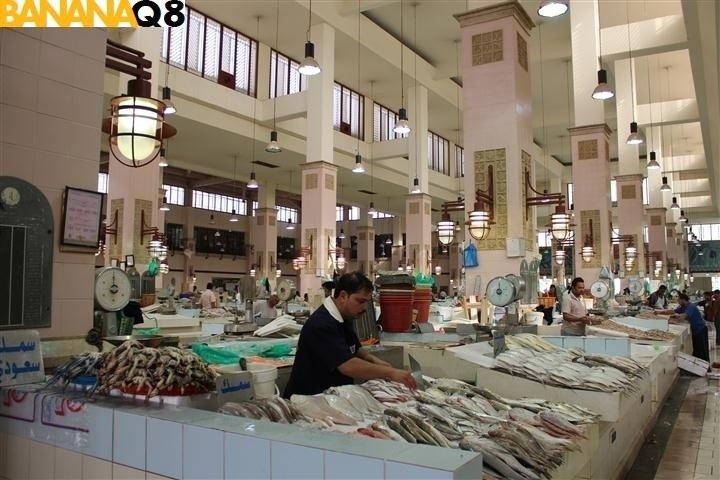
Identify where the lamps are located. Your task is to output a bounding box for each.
[104,37,168,168]
[206,0,705,285]
[96,209,119,257]
[156,60,178,118]
[139,187,172,275]
[156,140,170,168]
[189,268,197,283]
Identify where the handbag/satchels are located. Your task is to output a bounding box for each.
[703,294,720,322]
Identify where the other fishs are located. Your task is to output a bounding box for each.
[494,333,651,399]
[217,374,603,480]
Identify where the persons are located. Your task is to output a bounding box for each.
[282,272,418,400]
[560,277,593,336]
[647,285,720,373]
[198,282,217,309]
[543,284,559,299]
[254,295,280,315]
[321,279,334,300]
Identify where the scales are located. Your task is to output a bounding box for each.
[504,274,537,336]
[629,280,642,300]
[95,266,165,348]
[472,277,516,342]
[277,279,310,324]
[588,281,609,316]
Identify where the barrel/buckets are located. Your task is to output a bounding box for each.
[525,311,544,326]
[376,282,434,333]
[214,363,279,408]
